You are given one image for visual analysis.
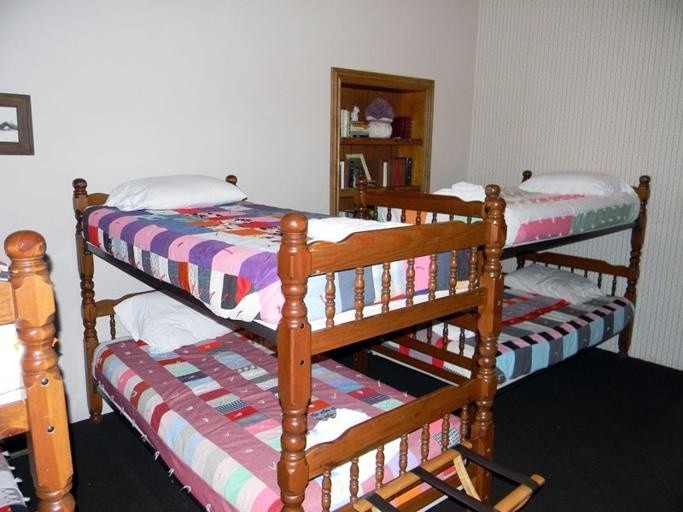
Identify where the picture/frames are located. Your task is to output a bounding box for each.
[0,92,35,155]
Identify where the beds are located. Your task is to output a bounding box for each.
[0,229,77,512]
[355,170,650,398]
[73,176,506,512]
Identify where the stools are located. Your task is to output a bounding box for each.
[353,441,544,512]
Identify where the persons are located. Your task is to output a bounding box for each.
[352,165,362,189]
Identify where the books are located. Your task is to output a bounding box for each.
[378,156,412,187]
[339,108,367,137]
[339,159,349,189]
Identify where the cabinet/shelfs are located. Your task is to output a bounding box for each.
[331,68,435,216]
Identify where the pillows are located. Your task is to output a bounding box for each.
[517,174,634,196]
[112,289,235,354]
[504,265,611,306]
[105,176,249,212]
